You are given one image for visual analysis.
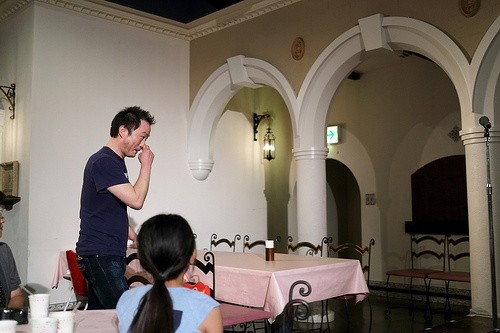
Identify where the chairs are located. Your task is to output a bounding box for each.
[384,233,470,323]
[64,234,375,333]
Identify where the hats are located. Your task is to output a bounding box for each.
[0,191,21,211]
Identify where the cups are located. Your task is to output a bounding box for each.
[28,293,75,333]
[266,248,274,261]
[0,320,17,333]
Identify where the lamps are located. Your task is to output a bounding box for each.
[253,113,276,162]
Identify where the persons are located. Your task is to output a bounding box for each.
[0,191,27,310]
[75,105,156,309]
[116,214,223,333]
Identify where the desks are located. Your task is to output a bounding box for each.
[51,246,370,333]
